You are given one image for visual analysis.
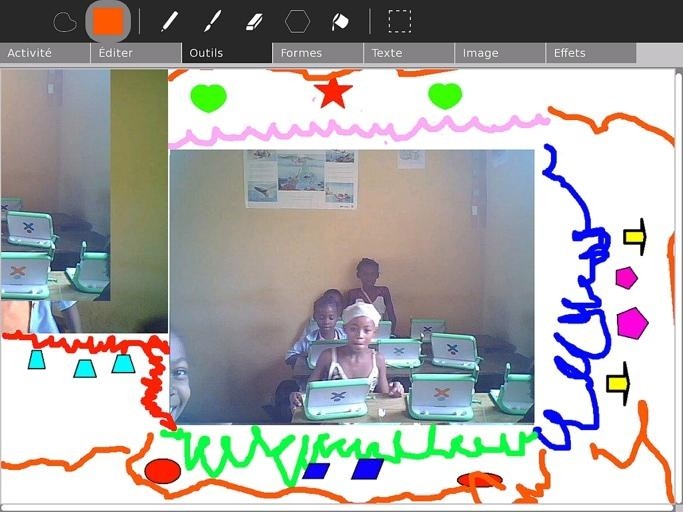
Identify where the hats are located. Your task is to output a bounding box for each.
[342,303,381,327]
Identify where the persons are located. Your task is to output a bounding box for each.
[342,257,399,339]
[300,289,345,338]
[170,325,190,423]
[289,298,404,414]
[284,297,348,368]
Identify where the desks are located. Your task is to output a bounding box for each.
[293,353,531,380]
[289,392,530,424]
[1,213,93,227]
[367,334,517,351]
[44,269,110,300]
[2,230,109,254]
